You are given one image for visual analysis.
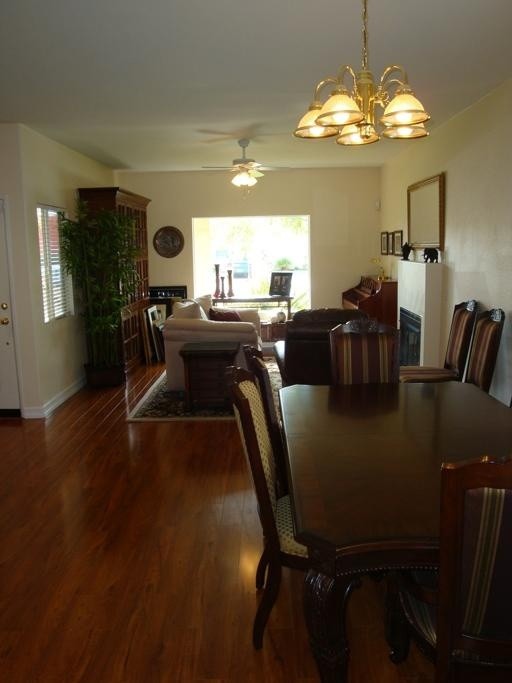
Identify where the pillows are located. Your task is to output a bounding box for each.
[172,301,201,318]
[209,308,218,319]
[214,311,239,321]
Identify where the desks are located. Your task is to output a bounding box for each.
[179,342,239,411]
[213,296,291,340]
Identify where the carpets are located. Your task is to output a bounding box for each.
[124,351,284,422]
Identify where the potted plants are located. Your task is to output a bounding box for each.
[59,199,141,388]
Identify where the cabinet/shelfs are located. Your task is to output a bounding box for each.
[76,186,151,375]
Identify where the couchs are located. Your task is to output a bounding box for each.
[163,297,262,394]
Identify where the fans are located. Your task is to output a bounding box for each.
[201,139,289,172]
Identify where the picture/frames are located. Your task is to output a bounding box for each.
[407,172,444,251]
[381,230,403,256]
[270,273,292,296]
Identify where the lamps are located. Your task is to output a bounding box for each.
[294,2,430,146]
[230,169,257,187]
